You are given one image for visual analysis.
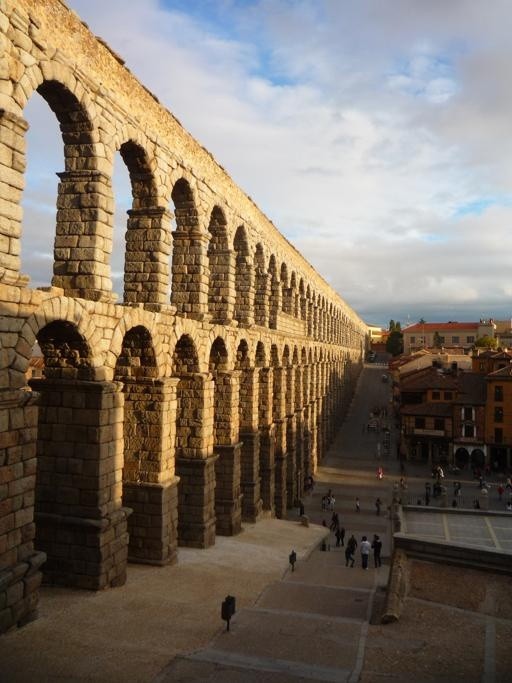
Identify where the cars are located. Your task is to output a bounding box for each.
[366,349,378,361]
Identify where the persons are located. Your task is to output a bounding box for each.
[294,461,512,568]
